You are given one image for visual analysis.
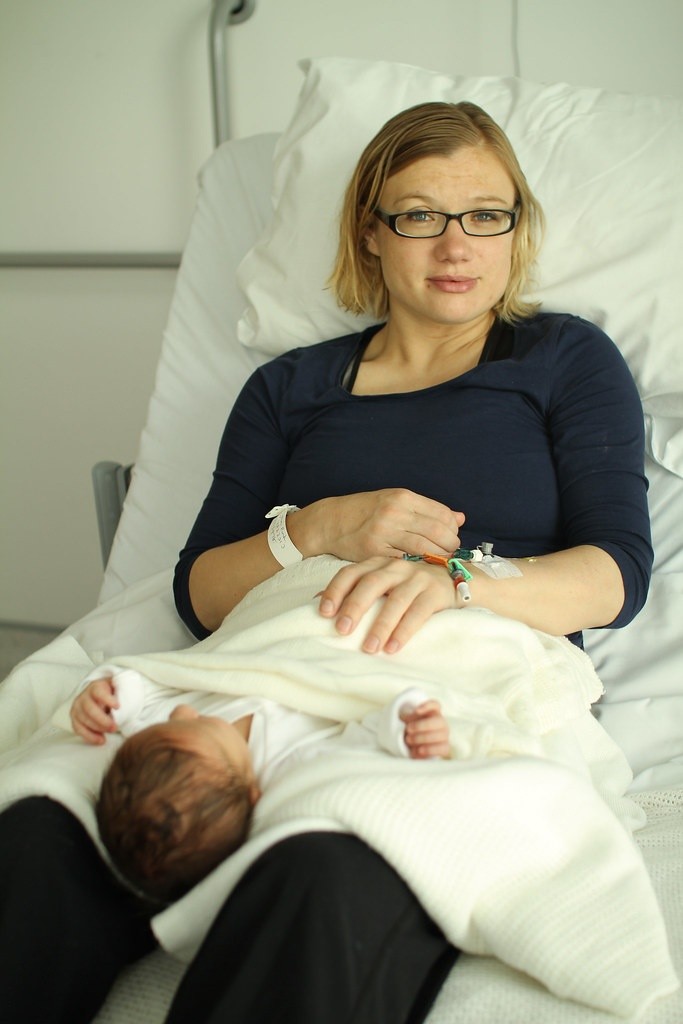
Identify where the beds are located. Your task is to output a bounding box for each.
[1,130,683,1024]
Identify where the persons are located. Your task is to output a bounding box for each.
[70,555,605,907]
[0,106,652,1024]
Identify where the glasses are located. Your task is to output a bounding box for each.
[369,200,525,237]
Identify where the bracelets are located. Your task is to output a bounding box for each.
[264,505,303,570]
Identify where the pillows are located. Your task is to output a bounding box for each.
[236,56,683,480]
